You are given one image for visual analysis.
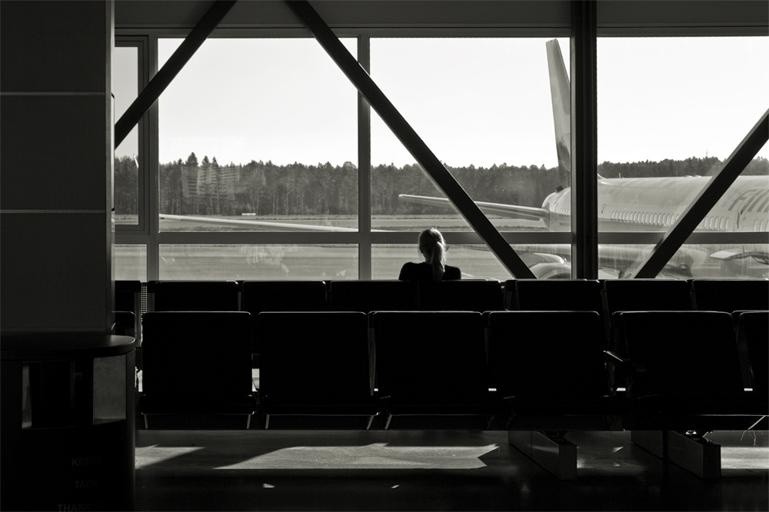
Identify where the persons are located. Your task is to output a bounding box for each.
[398,227,461,282]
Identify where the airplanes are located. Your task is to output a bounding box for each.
[398,40,769,264]
